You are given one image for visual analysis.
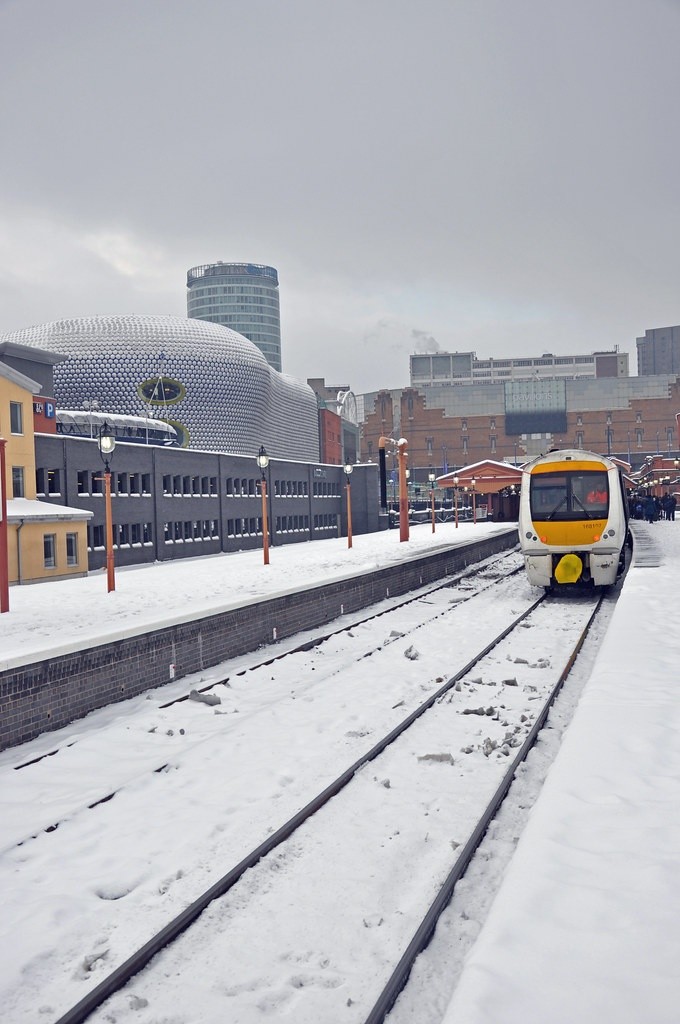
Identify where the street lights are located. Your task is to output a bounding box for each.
[82,399,98,440]
[513,441,517,467]
[93,422,118,591]
[406,468,410,516]
[471,475,477,525]
[453,473,459,527]
[442,444,446,475]
[344,458,353,549]
[146,410,153,446]
[387,450,399,511]
[643,477,663,497]
[428,469,435,533]
[256,445,271,565]
[389,479,395,527]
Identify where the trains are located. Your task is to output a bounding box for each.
[517,449,631,597]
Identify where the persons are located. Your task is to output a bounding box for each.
[627,492,677,523]
[587,482,607,504]
